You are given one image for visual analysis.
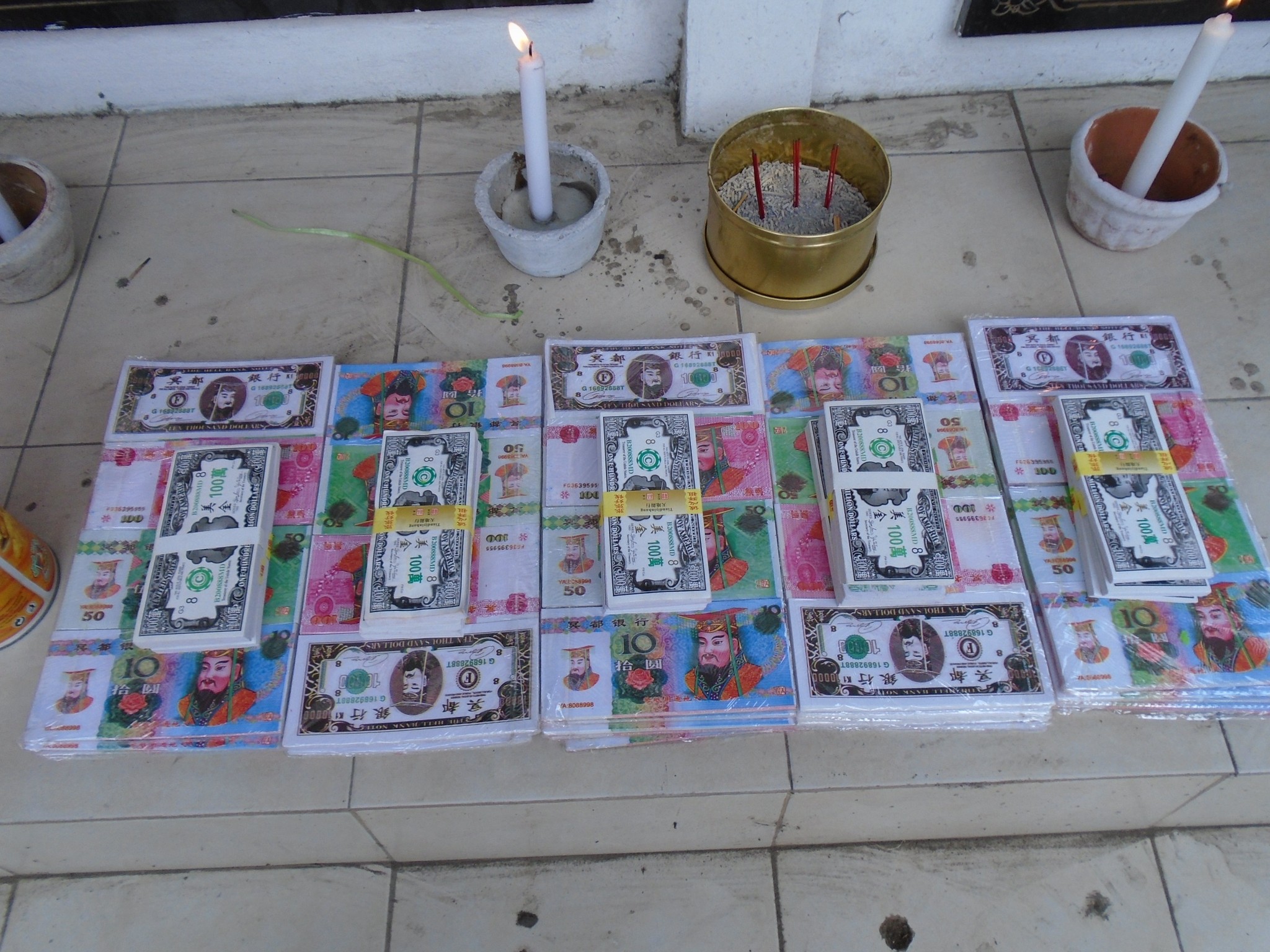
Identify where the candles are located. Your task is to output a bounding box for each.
[507,22,554,221]
[1120,0,1241,200]
[0,192,25,242]
